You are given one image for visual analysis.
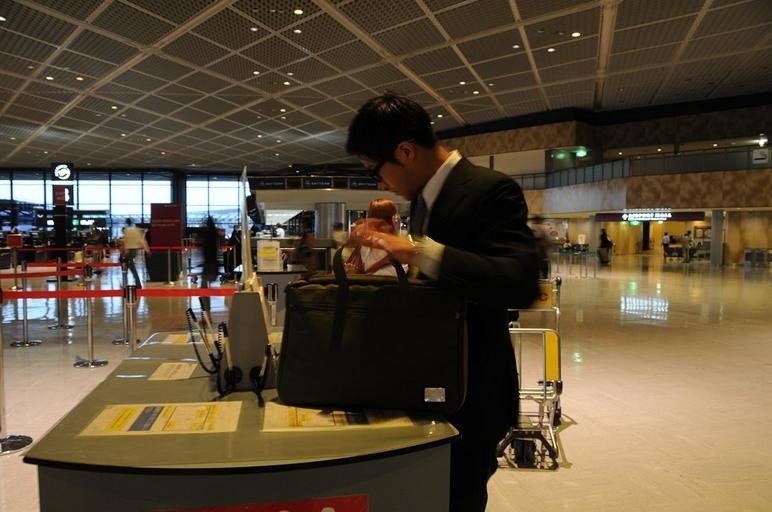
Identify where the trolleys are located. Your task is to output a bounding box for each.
[497,275,566,472]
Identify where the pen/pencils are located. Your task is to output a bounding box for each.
[220,323,234,373]
[188,308,217,367]
[259,344,270,377]
[197,298,213,336]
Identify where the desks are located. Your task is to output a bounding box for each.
[18,332,460,512]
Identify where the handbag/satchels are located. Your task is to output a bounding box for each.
[276,275,469,421]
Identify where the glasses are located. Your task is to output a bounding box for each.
[368,138,414,184]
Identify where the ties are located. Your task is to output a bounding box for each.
[410,193,428,235]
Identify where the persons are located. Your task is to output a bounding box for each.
[118,219,148,290]
[200,199,407,280]
[600,229,613,253]
[682,230,697,263]
[662,232,672,257]
[332,91,547,512]
